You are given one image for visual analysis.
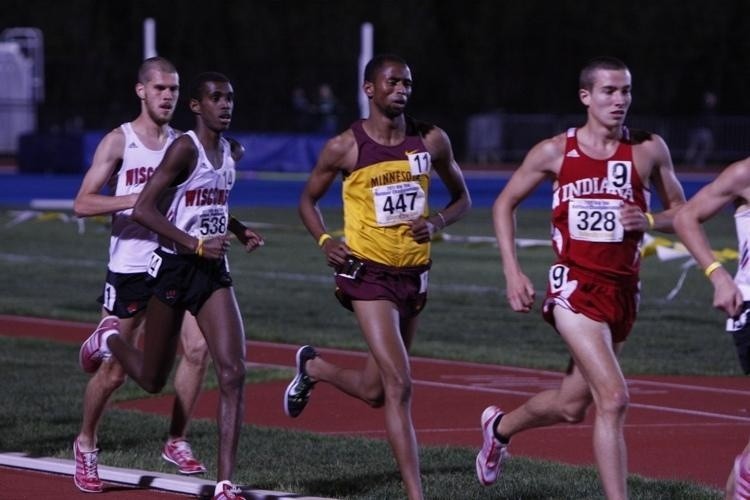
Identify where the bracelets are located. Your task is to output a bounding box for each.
[704,261,722,276]
[229,214,235,221]
[644,212,654,236]
[437,212,448,230]
[196,239,204,257]
[318,234,333,248]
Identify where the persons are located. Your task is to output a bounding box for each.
[475,58,687,500]
[72,56,207,494]
[672,158,750,500]
[290,86,315,128]
[79,70,265,500]
[694,93,718,164]
[283,52,473,500]
[314,84,341,136]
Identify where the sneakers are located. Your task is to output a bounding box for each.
[734,453,750,500]
[79,316,120,372]
[212,484,246,500]
[476,406,512,487]
[73,437,104,493]
[284,345,320,418]
[162,438,208,473]
